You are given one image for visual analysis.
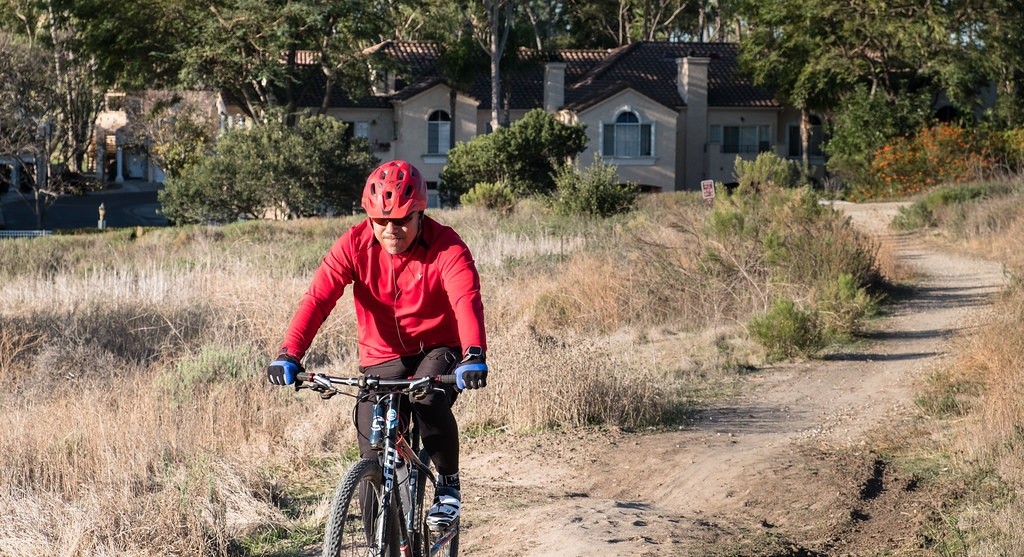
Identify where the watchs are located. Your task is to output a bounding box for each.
[464,345,484,357]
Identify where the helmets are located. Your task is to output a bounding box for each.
[361,161,428,218]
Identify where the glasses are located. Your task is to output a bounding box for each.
[371,210,420,227]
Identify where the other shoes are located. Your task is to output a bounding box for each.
[425,467,461,531]
[367,548,379,557]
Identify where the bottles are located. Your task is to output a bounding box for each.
[395,456,413,516]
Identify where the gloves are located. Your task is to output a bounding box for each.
[454,355,488,394]
[267,354,306,391]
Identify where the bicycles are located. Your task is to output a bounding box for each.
[294,372,460,557]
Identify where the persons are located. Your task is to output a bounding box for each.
[267,160,488,557]
